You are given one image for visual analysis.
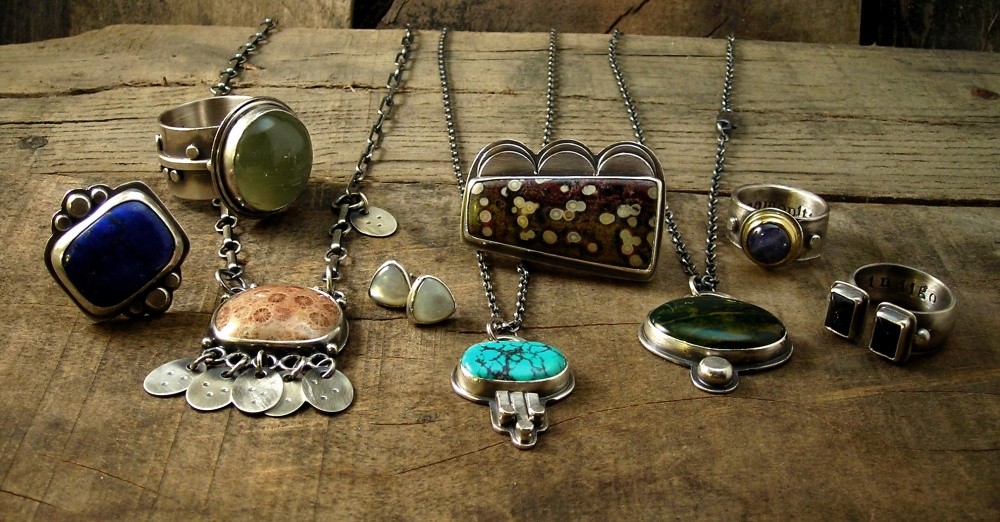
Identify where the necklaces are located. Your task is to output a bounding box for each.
[142,18,418,416]
[607,28,792,393]
[433,22,576,449]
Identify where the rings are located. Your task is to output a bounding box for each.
[823,262,957,364]
[155,94,314,220]
[724,186,831,268]
[42,181,191,325]
